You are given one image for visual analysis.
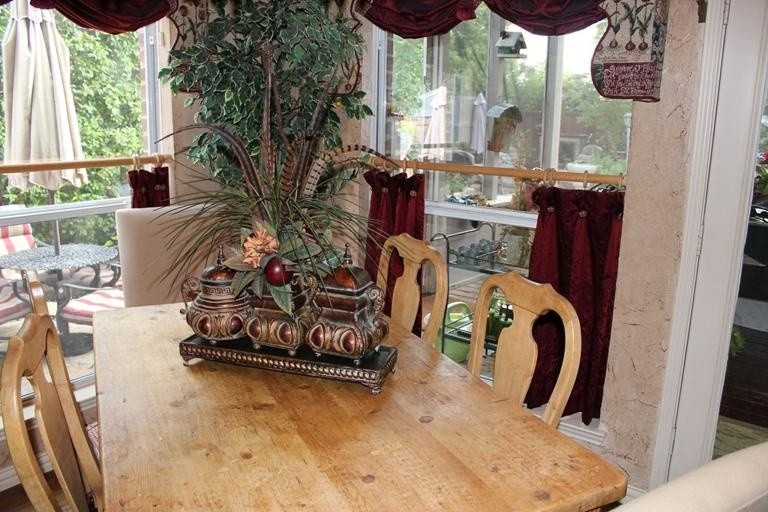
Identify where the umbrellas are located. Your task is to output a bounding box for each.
[425,85,449,159]
[469,93,487,164]
[0,1,93,252]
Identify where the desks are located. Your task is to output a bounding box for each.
[101,255,121,288]
[0,243,118,356]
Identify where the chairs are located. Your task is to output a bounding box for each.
[24,270,102,494]
[92,299,628,511]
[0,284,32,355]
[57,263,125,343]
[0,203,57,307]
[374,232,449,344]
[467,271,582,429]
[0,313,104,512]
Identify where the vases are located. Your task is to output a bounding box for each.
[178,241,398,396]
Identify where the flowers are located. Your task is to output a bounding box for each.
[149,0,419,316]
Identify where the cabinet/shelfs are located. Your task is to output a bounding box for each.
[430,222,529,354]
